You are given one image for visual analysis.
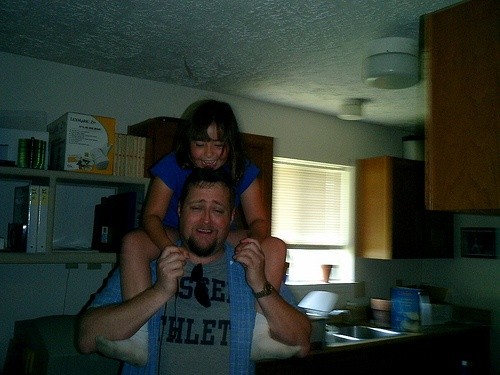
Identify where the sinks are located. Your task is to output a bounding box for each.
[332,324,405,341]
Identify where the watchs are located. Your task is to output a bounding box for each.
[252,281,272,298]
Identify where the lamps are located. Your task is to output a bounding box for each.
[338,100,362,121]
[360,37,419,89]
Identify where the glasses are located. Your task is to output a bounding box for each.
[190,263,211,307]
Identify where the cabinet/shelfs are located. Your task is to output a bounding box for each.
[357,156,454,260]
[420,0,500,214]
[0,117,275,264]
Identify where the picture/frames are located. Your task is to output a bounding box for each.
[460,227,495,258]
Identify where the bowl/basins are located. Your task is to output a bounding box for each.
[346,300,369,323]
[421,304,451,324]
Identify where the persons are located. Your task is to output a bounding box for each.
[77,166,312,375]
[95,99,301,367]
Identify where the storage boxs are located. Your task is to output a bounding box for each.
[371,284,421,331]
[46,112,116,175]
